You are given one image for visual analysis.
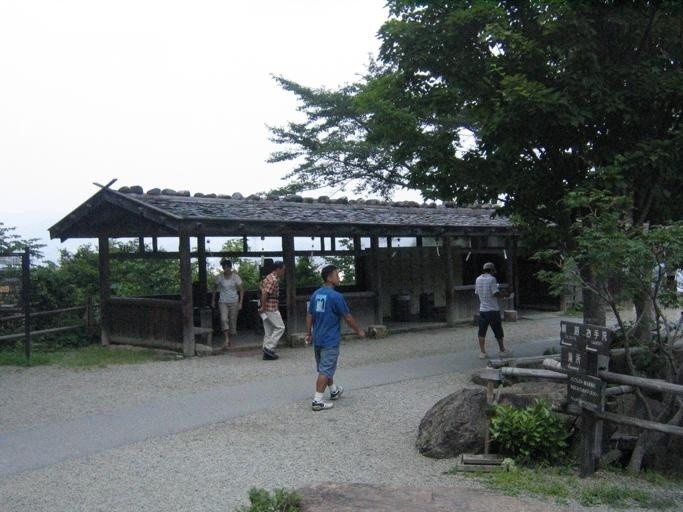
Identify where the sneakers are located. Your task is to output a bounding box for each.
[312,401,333,410]
[480,352,488,359]
[331,385,343,400]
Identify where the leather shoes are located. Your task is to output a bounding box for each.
[263,348,278,360]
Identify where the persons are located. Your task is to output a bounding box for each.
[305,265,364,411]
[259,260,286,359]
[210,260,245,348]
[475,262,512,358]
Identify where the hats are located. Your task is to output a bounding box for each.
[483,263,496,273]
